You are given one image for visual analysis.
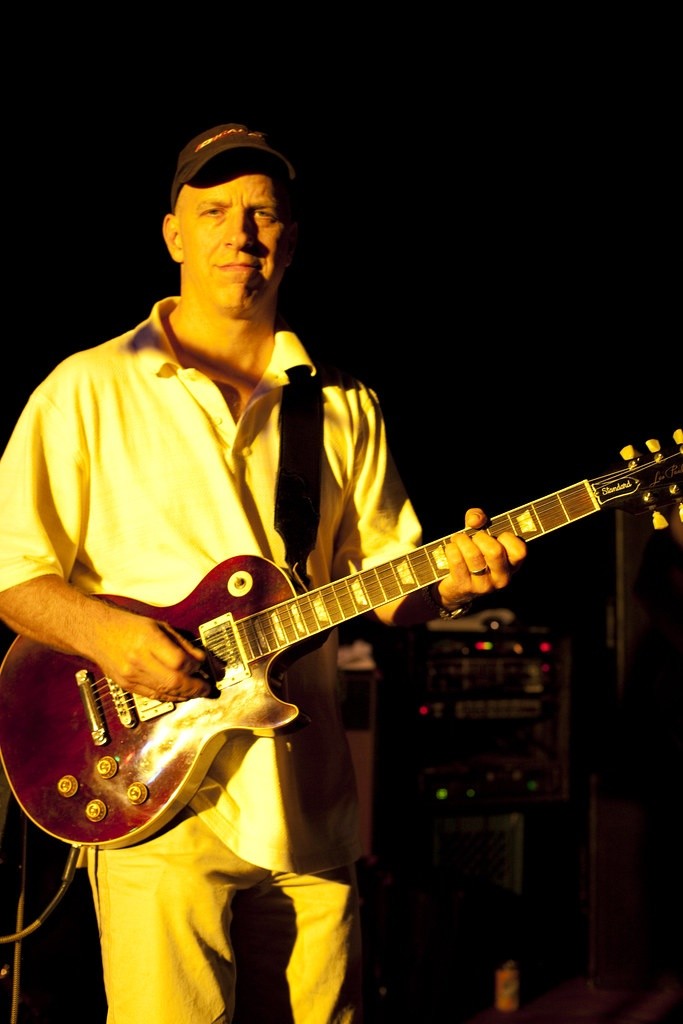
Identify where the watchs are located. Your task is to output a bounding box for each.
[420,586,480,619]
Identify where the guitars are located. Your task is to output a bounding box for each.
[0,426,682,853]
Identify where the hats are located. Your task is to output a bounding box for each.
[171,122,296,214]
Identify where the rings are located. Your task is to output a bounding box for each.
[468,564,489,575]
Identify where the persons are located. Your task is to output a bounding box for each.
[0,125,526,1024]
